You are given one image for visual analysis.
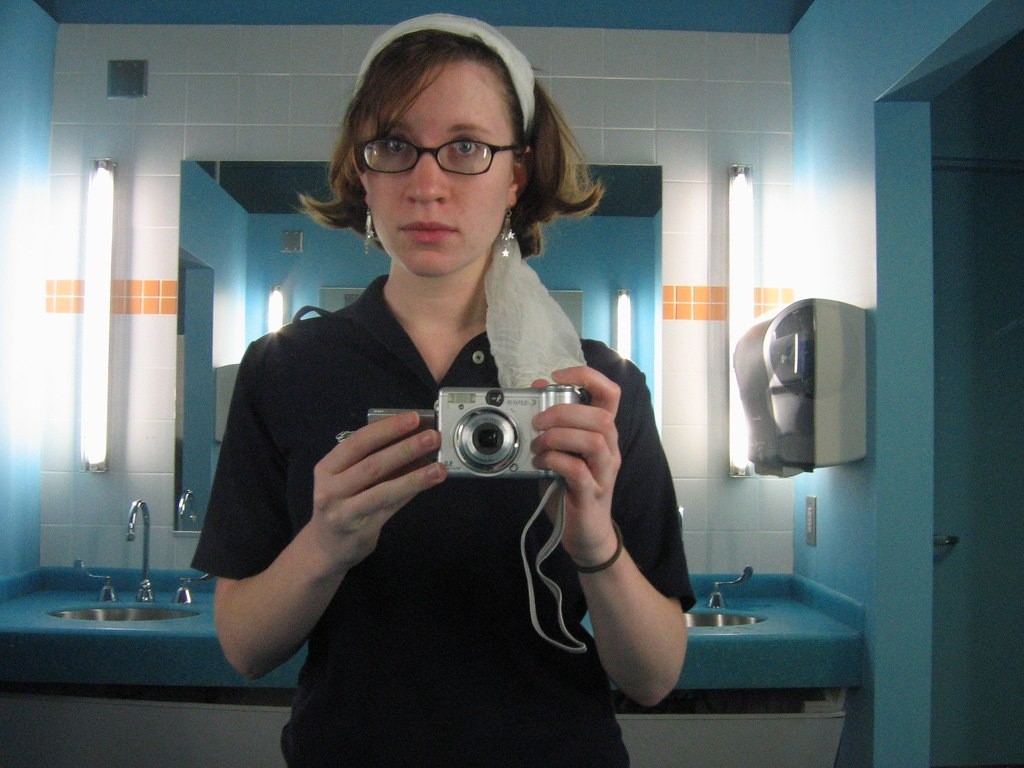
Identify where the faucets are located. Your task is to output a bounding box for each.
[179,489,198,531]
[126,498,156,603]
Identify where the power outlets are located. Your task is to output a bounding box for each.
[805,496,817,545]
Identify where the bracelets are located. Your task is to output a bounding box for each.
[570,518,621,575]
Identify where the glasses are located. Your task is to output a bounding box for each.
[354,136,522,174]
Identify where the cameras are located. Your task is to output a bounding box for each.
[433,384,583,481]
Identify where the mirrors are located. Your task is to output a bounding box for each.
[175,159,662,537]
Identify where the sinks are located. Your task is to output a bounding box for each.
[49,607,201,621]
[683,612,765,627]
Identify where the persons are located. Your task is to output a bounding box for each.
[189,12,697,763]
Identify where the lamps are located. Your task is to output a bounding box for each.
[728,164,754,480]
[78,156,116,472]
[269,286,285,335]
[618,290,631,361]
